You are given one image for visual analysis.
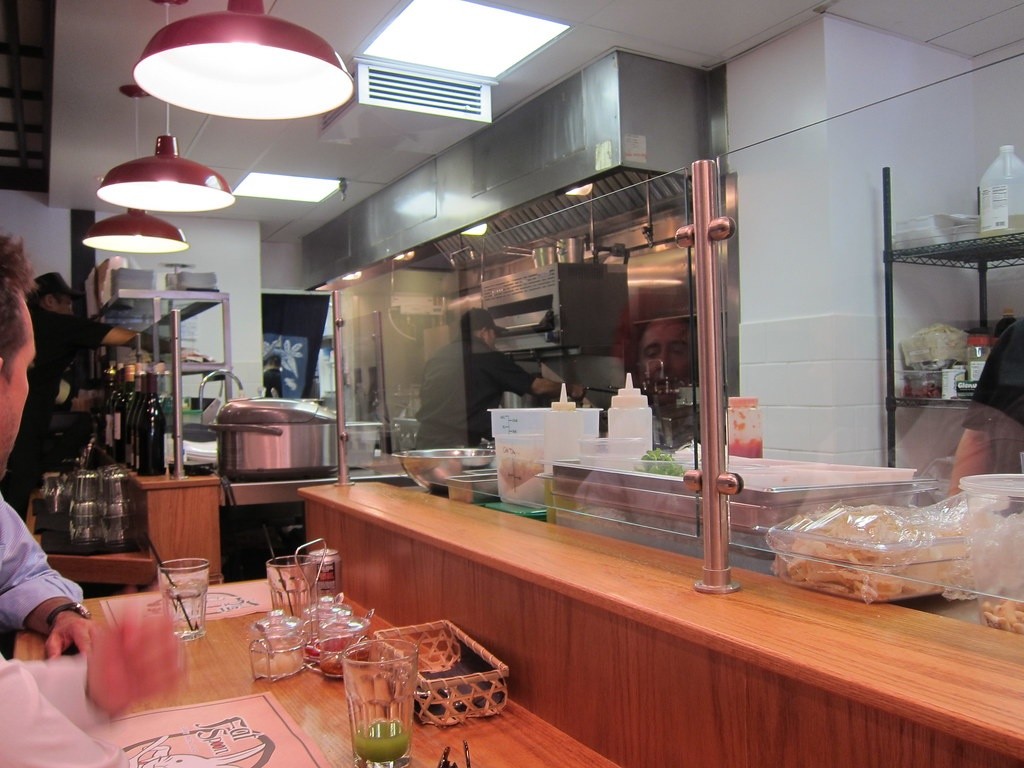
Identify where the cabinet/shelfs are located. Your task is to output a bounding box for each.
[83,285,240,486]
[873,156,1024,503]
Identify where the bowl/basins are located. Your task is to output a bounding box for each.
[385,447,496,491]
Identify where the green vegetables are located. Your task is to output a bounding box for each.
[635,448,684,478]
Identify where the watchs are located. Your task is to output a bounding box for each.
[46,600,90,624]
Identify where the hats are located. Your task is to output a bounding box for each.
[23,271,82,301]
[457,306,509,336]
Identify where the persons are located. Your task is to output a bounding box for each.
[413,306,586,454]
[943,316,1023,518]
[1,228,193,768]
[634,309,700,438]
[262,353,285,399]
[3,272,178,658]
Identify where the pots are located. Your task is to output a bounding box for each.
[202,397,340,480]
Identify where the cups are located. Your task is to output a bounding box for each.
[44,461,137,547]
[343,637,420,768]
[531,236,584,267]
[266,555,319,615]
[158,556,211,644]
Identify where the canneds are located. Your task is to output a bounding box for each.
[308,549,343,597]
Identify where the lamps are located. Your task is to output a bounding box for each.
[80,84,193,254]
[86,0,240,215]
[129,0,364,123]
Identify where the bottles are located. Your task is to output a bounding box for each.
[607,371,653,457]
[100,362,165,477]
[545,383,581,474]
[724,395,764,459]
[994,309,1016,348]
[965,333,989,385]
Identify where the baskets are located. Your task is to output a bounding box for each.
[372,617,511,728]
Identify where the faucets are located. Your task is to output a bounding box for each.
[197,367,245,418]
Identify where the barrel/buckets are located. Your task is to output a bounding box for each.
[978,144,1024,236]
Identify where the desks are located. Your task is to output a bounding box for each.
[11,574,635,768]
